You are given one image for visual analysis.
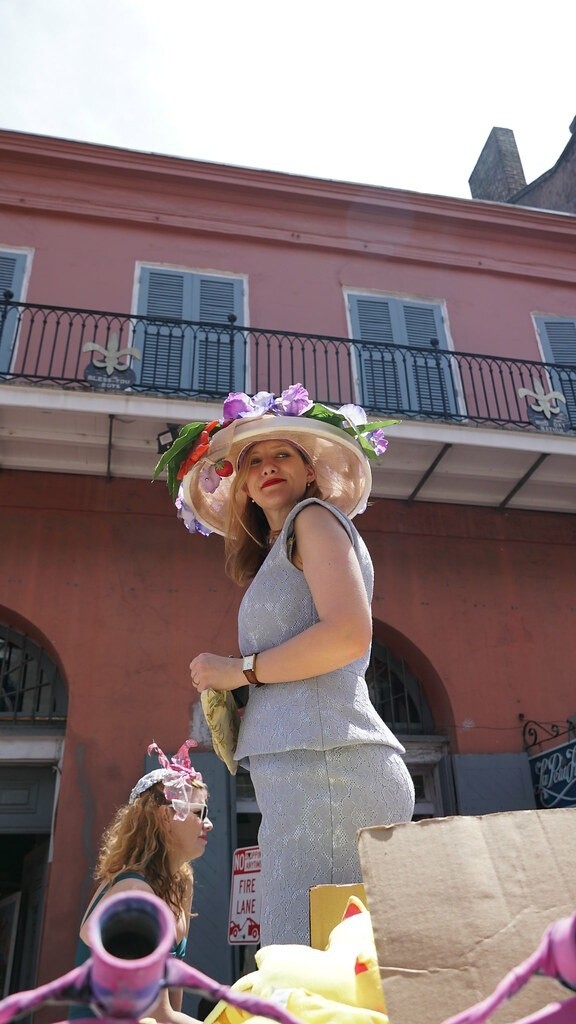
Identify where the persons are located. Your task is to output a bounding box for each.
[192,400,415,960]
[81,766,216,1024]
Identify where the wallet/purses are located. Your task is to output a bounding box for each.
[201,688,242,777]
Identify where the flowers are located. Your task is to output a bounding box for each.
[150,379,404,536]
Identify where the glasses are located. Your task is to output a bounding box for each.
[165,802,208,822]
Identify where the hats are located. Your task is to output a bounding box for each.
[182,417,373,545]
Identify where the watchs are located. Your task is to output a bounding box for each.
[240,651,264,688]
